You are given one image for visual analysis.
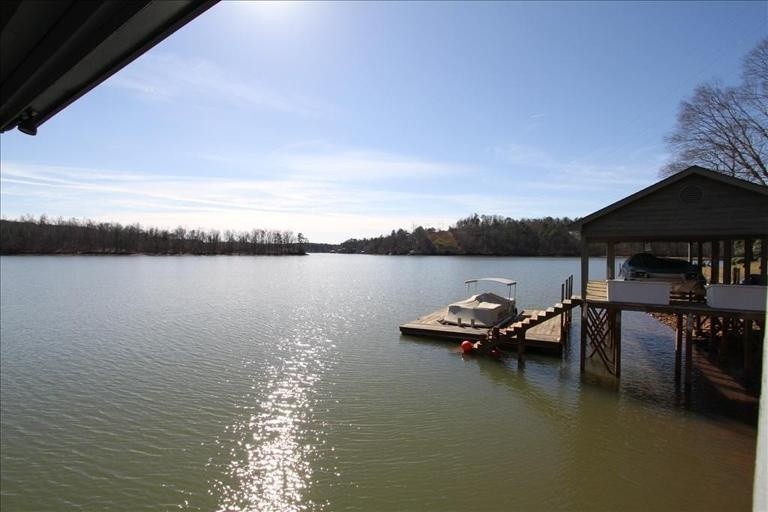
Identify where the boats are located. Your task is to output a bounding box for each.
[442,275,518,327]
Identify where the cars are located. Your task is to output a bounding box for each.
[620,252,706,287]
[739,273,762,287]
